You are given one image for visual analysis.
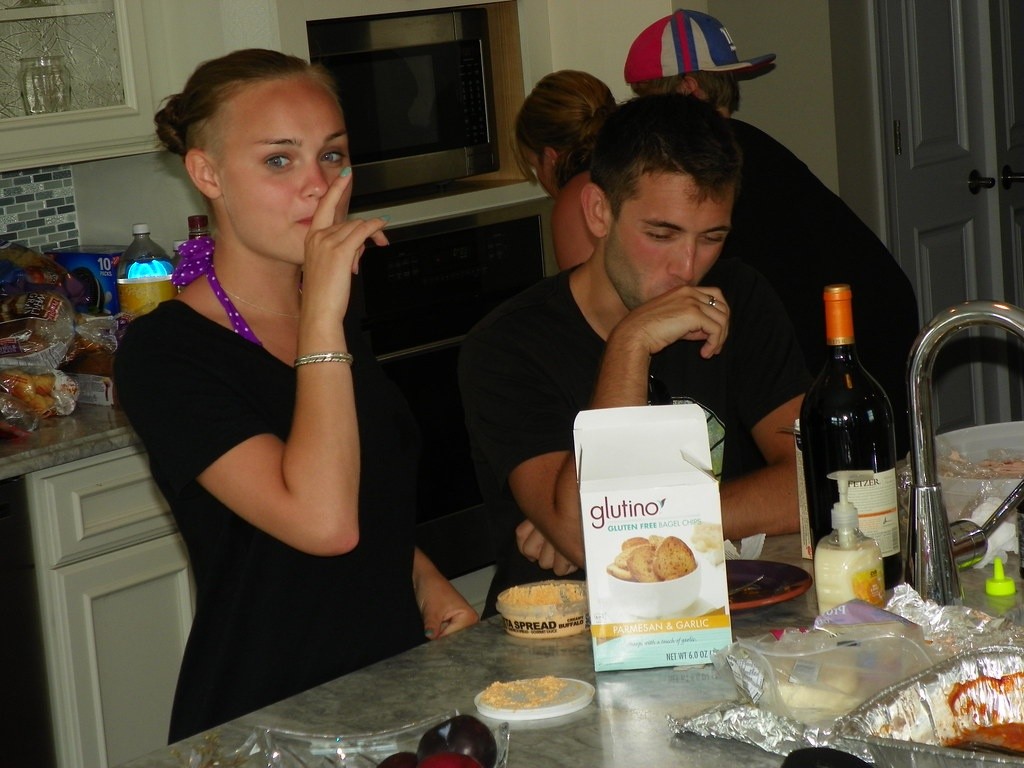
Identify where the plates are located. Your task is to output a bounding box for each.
[725,558,813,613]
[223,711,511,768]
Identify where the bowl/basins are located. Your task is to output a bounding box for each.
[497,578,588,640]
[607,561,701,618]
[906,418,1023,523]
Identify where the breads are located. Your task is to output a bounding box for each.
[607,536,696,583]
[0,289,104,419]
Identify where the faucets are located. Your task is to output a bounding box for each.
[904,299,1024,607]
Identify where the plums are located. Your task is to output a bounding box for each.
[374,713,498,768]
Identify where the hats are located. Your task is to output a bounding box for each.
[625,9,776,82]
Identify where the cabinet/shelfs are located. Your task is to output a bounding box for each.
[0,0,157,175]
[26,445,196,768]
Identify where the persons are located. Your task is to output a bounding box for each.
[458,90,824,624]
[113,48,478,745]
[510,68,920,464]
[623,8,778,120]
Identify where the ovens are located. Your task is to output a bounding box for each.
[344,216,544,529]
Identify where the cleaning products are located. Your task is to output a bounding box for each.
[813,470,886,617]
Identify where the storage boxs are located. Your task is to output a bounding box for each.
[574,403,733,671]
[60,352,116,406]
[45,244,129,315]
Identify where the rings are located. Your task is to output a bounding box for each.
[709,295,717,305]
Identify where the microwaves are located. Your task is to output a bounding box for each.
[305,8,500,205]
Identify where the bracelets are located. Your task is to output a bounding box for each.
[293,351,354,366]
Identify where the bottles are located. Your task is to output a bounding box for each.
[18,56,71,116]
[187,214,213,240]
[171,238,187,268]
[799,282,905,591]
[115,222,178,321]
